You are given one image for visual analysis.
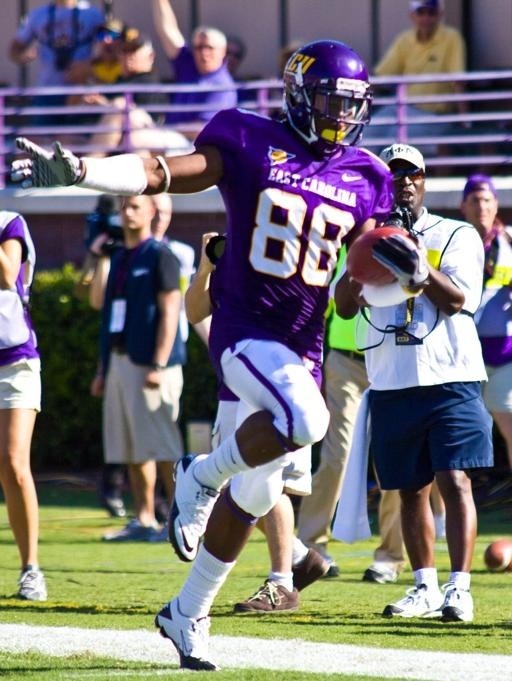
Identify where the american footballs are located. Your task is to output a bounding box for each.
[485,542,512,572]
[347,226,415,287]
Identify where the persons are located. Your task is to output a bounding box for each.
[75,191,214,543]
[0,208,51,605]
[451,177,511,542]
[15,0,471,185]
[295,240,405,581]
[8,39,433,670]
[187,229,329,616]
[337,142,493,626]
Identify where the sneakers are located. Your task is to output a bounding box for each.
[440,589,474,623]
[434,511,447,540]
[291,547,331,590]
[17,571,48,602]
[167,453,219,563]
[98,481,172,543]
[362,561,399,584]
[307,543,340,579]
[233,587,300,614]
[382,588,444,619]
[153,600,220,672]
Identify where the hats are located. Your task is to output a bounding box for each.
[378,144,425,175]
[120,26,146,50]
[409,0,446,15]
[462,175,497,200]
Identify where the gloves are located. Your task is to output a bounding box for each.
[11,136,81,190]
[371,233,431,290]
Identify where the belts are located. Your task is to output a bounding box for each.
[330,345,371,365]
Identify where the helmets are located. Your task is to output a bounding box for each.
[284,40,373,149]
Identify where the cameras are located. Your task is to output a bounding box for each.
[205,235,226,264]
[384,204,413,237]
